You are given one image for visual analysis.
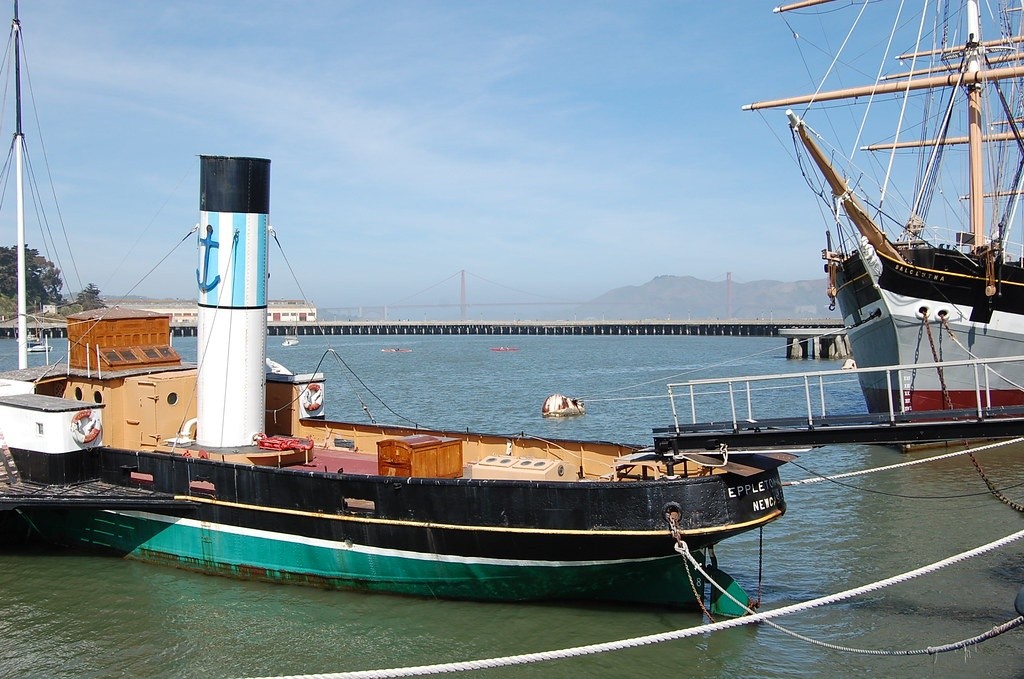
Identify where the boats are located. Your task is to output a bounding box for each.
[16,333,53,354]
[0,0,800,618]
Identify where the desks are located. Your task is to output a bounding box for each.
[612,447,691,481]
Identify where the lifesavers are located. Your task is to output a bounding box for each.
[303,383,323,411]
[71,410,101,444]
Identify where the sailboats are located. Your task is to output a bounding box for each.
[741,0,1023,413]
